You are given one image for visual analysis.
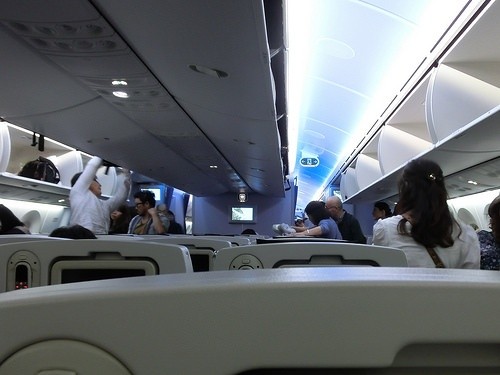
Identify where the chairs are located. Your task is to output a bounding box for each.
[0,232,500,375]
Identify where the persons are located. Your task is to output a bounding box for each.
[48,224,97,239]
[242,229,257,235]
[110,191,184,234]
[325,196,366,244]
[290,200,342,240]
[293,216,318,232]
[371,159,481,270]
[393,202,399,216]
[0,203,31,236]
[475,195,500,271]
[469,223,479,231]
[67,155,130,235]
[372,201,392,220]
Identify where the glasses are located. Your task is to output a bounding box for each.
[135,203,141,208]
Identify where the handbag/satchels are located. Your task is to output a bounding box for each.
[18,156,60,184]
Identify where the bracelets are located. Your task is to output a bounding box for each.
[306,229,310,235]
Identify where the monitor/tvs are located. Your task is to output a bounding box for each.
[228,206,257,224]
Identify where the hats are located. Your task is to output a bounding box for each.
[272,223,297,236]
